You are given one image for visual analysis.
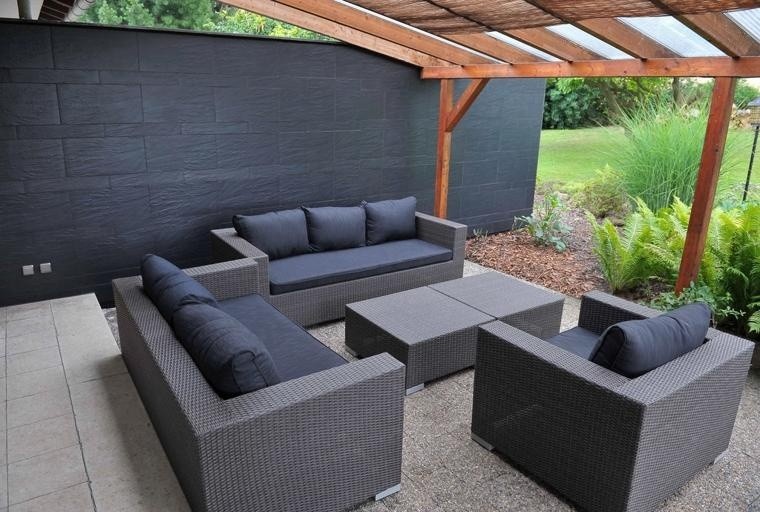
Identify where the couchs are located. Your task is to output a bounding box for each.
[209,211,468,328]
[112,260,405,512]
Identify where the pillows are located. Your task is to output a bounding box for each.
[301,205,367,251]
[362,194,419,246]
[232,207,313,260]
[165,295,282,397]
[589,300,711,376]
[140,253,219,316]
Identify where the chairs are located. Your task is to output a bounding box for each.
[471,289,756,512]
